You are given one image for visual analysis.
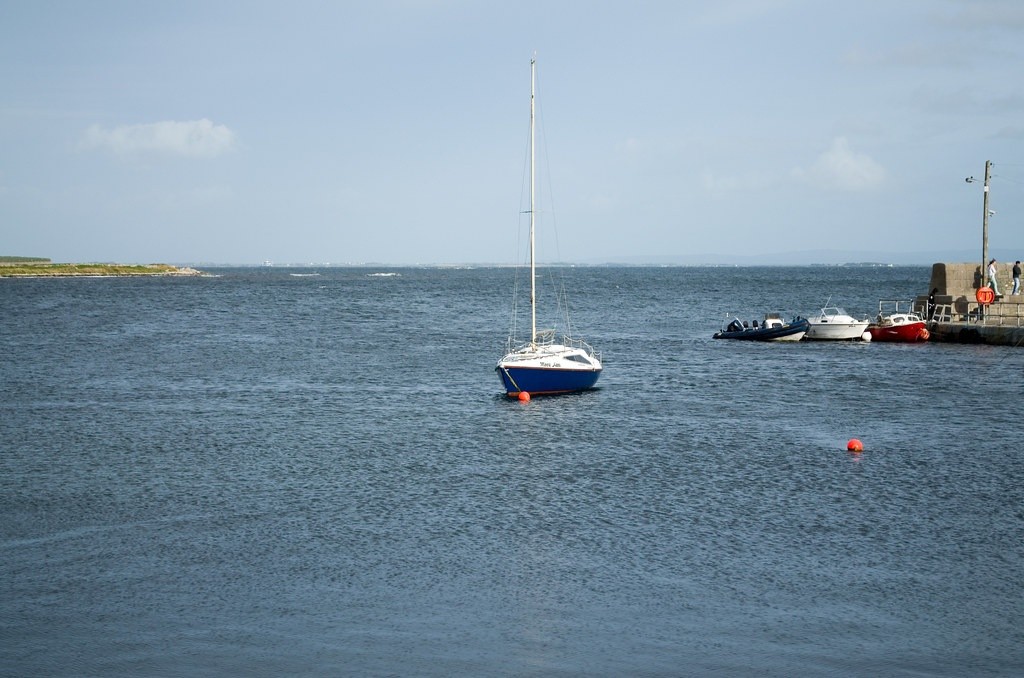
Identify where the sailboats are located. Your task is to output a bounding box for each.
[496,55,601,399]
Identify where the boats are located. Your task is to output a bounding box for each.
[865,299,930,342]
[803,294,869,341]
[712,314,811,342]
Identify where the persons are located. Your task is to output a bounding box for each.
[875,310,884,325]
[986,258,1003,296]
[1012,261,1022,296]
[927,287,939,323]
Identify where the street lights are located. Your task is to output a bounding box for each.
[966,178,990,315]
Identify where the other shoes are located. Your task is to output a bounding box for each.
[1012,292,1020,296]
[996,292,1002,296]
